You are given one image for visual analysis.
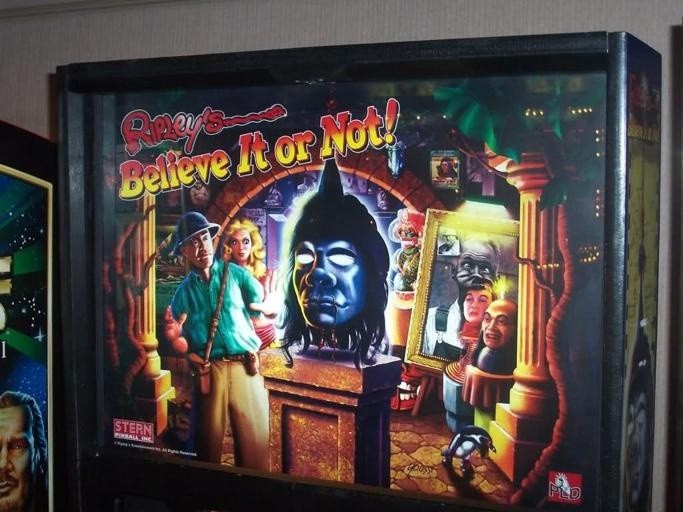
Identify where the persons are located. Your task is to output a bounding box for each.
[471,298,518,366]
[274,196,391,371]
[421,238,502,361]
[222,218,277,350]
[458,284,497,323]
[440,158,457,177]
[623,334,656,512]
[164,212,283,472]
[0,391,47,512]
[439,228,459,254]
